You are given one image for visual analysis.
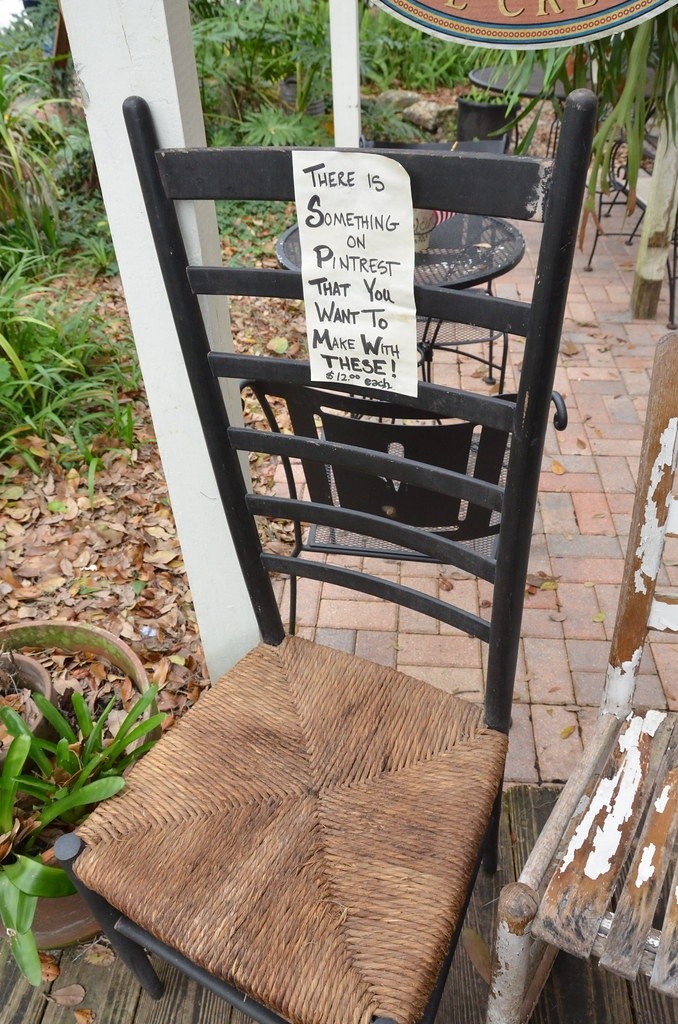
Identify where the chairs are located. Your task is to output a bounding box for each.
[584,96,678,333]
[54,95,593,1024]
[239,374,568,637]
[358,101,529,270]
[483,330,678,1024]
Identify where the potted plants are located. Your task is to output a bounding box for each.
[0,621,166,988]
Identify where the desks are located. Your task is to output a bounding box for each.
[469,67,576,158]
[276,210,526,426]
